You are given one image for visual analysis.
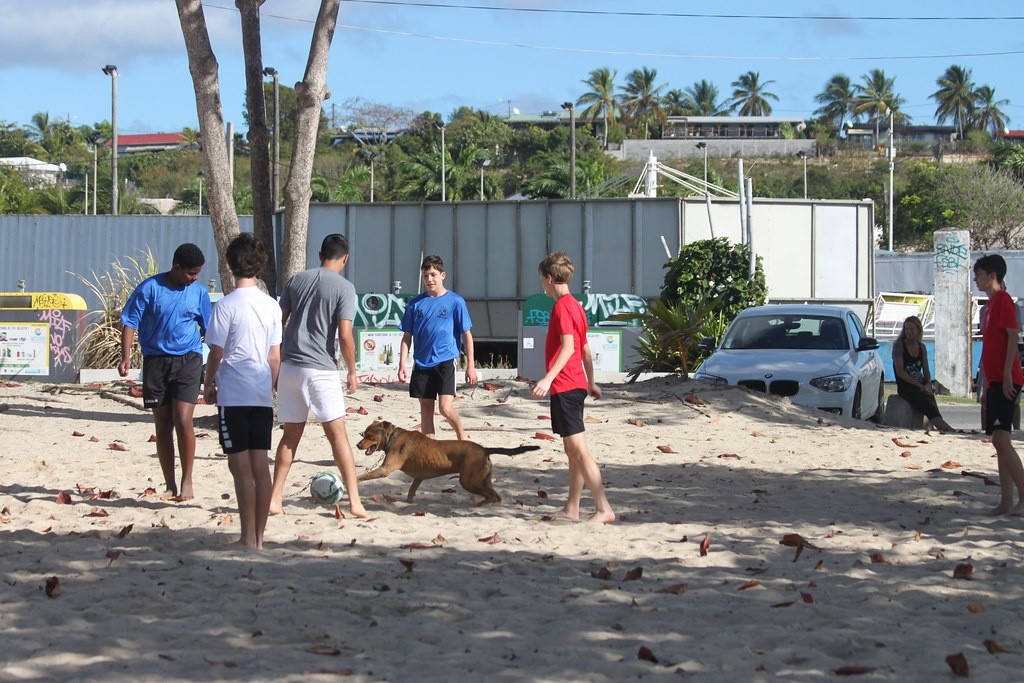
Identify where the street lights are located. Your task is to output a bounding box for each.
[561,102,575,199]
[696,142,707,191]
[102,64,118,215]
[796,150,807,199]
[263,66,280,212]
[431,119,445,203]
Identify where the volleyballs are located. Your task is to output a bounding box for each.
[310,470,344,504]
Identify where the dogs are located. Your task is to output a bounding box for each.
[355,421,541,507]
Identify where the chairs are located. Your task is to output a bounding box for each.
[756,322,786,347]
[820,320,845,349]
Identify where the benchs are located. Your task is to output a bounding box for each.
[786,330,822,348]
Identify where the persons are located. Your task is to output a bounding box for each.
[118,243,213,499]
[398,255,477,440]
[204,232,282,549]
[270,233,371,518]
[892,316,955,432]
[530,252,616,522]
[973,254,1024,515]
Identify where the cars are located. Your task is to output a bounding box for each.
[693,303,884,425]
